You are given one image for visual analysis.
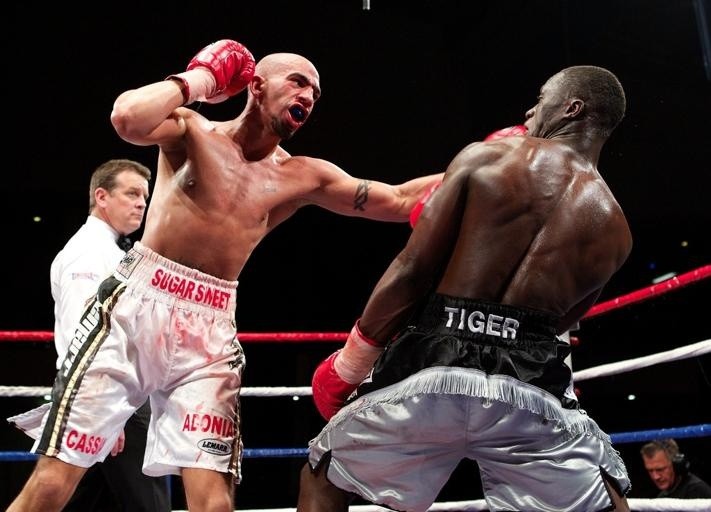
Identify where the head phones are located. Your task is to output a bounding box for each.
[652,439,690,475]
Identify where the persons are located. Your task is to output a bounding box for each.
[640,435,710,498]
[296,66,637,511]
[6,37,528,512]
[50,160,172,508]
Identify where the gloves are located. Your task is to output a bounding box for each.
[486,126,526,140]
[410,181,441,229]
[165,39,255,106]
[313,320,385,420]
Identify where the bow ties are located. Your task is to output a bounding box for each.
[118,235,131,250]
